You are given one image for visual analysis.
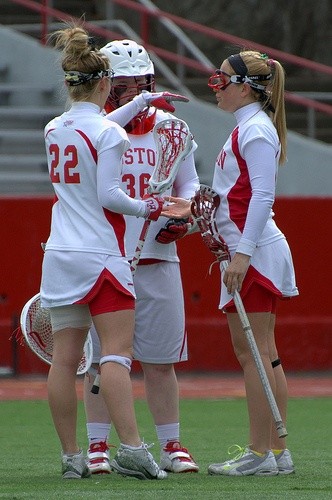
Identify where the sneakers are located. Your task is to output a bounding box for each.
[271,448,296,475]
[110,437,167,480]
[61,448,92,480]
[159,440,200,473]
[86,439,111,475]
[208,444,279,476]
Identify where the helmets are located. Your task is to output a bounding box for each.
[99,39,155,79]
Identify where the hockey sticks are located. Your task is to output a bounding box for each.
[20,292,95,375]
[189,184,289,439]
[91,118,193,395]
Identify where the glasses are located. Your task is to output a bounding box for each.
[113,83,148,95]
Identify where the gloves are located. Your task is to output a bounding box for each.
[139,90,190,113]
[142,193,164,221]
[155,216,193,242]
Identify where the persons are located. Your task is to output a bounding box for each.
[160,50,296,477]
[39,28,199,481]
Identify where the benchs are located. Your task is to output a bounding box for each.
[0,81,213,195]
